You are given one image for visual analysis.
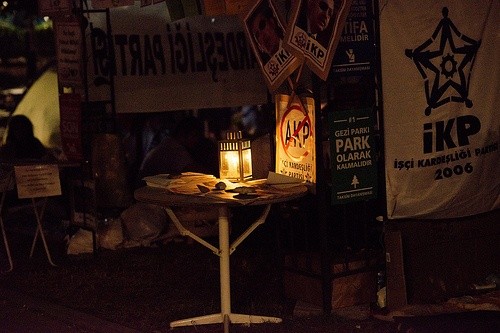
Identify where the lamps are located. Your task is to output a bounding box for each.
[218,131,253,183]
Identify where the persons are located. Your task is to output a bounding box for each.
[253,11,284,56]
[298,0,334,48]
[0,115,58,161]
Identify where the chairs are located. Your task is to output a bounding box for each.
[0,160,62,272]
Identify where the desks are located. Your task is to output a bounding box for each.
[134,185,307,333]
